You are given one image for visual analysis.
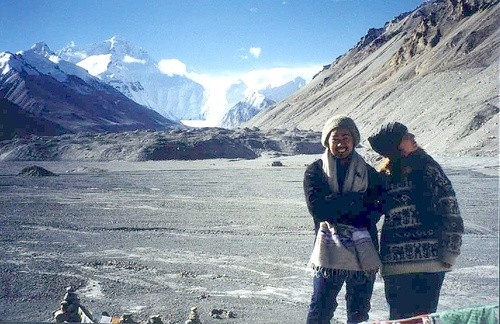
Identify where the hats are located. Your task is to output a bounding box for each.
[368,122,407,158]
[321,116,360,147]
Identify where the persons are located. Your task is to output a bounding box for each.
[368,122,464,321]
[303,116,380,324]
